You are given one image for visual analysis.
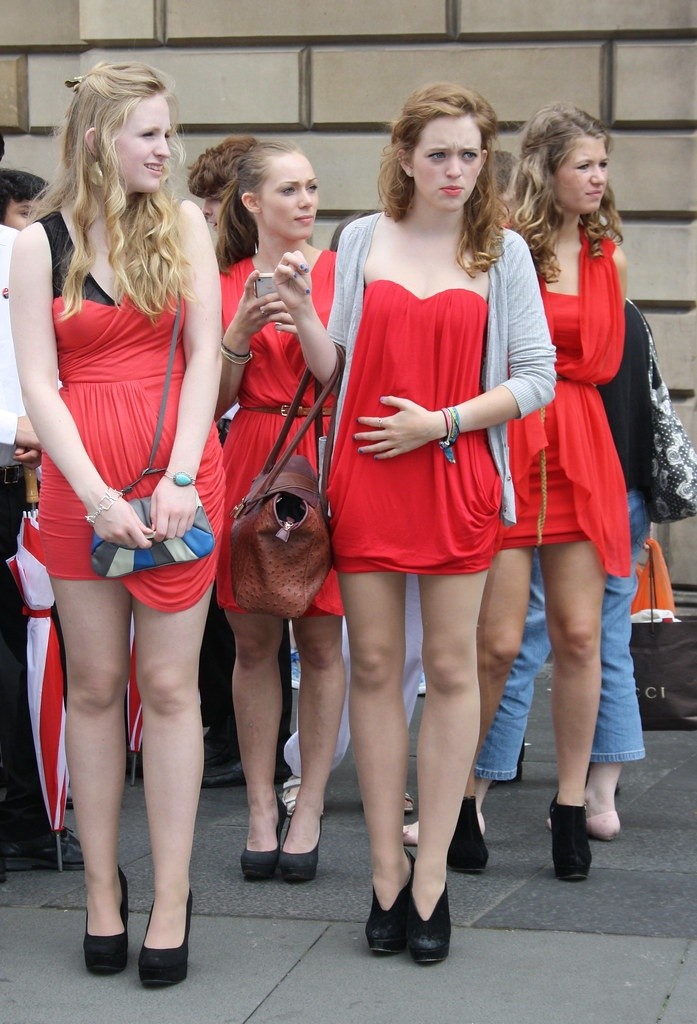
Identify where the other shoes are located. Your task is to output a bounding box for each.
[201,727,293,788]
[586,764,620,795]
[489,763,525,788]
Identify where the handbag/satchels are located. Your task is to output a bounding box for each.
[230,342,345,619]
[625,297,697,524]
[629,538,697,731]
[90,487,215,578]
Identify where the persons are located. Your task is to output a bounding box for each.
[0,131,87,872]
[187,134,296,783]
[272,82,558,966]
[205,143,338,884]
[277,147,697,847]
[449,107,629,884]
[10,60,224,987]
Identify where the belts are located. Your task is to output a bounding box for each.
[0,464,24,485]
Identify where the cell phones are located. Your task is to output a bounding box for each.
[254,273,277,298]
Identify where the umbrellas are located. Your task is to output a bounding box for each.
[126,609,142,784]
[10,463,69,870]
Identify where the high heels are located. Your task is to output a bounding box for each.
[83,865,128,972]
[365,848,416,953]
[406,859,451,963]
[447,794,489,871]
[138,888,193,984]
[240,792,287,879]
[278,808,324,882]
[549,792,592,879]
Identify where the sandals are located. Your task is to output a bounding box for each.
[404,793,414,811]
[282,775,324,818]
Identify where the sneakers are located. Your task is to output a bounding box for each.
[0,826,85,883]
[403,813,486,845]
[546,811,620,841]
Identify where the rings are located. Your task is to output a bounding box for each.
[378,418,383,427]
[261,309,267,317]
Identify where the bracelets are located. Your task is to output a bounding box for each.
[439,407,462,463]
[164,469,200,488]
[80,486,127,524]
[221,339,253,366]
